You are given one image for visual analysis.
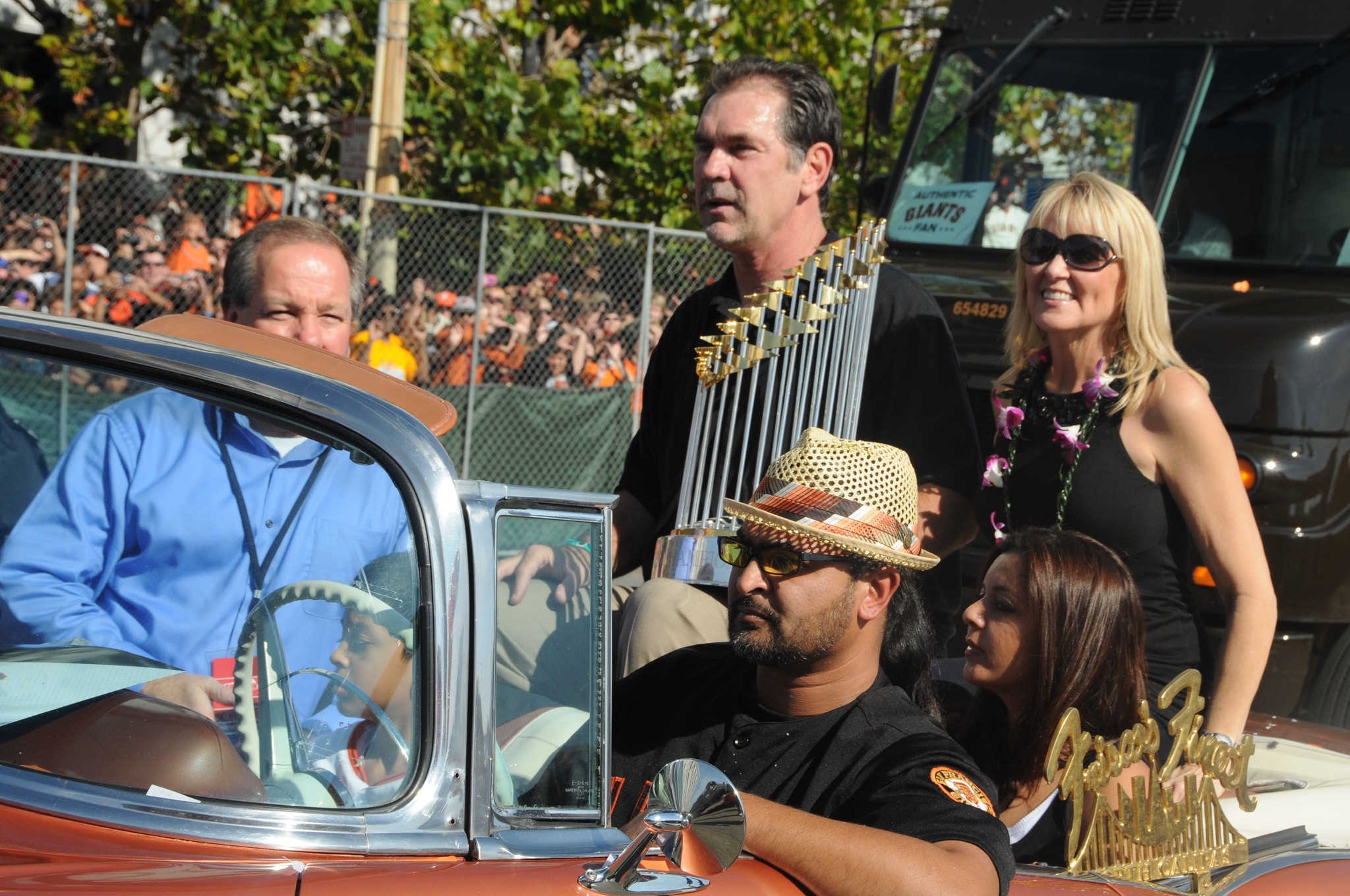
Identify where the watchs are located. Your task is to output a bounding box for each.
[1201,730,1237,748]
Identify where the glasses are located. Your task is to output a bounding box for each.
[717,534,887,576]
[1018,228,1125,271]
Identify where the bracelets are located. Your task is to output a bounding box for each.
[563,538,590,553]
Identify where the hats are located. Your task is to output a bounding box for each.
[722,426,941,570]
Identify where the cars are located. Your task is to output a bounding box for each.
[0,312,1348,896]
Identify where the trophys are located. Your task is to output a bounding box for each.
[651,216,893,587]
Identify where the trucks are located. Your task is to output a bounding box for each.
[856,0,1350,730]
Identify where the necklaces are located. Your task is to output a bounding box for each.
[983,355,1122,546]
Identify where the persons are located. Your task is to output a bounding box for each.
[972,171,1278,807]
[963,527,1160,870]
[1137,145,1235,260]
[351,269,681,412]
[497,55,983,708]
[718,795,739,816]
[981,171,1029,249]
[519,426,1015,896]
[304,551,519,807]
[0,164,283,393]
[0,217,411,765]
